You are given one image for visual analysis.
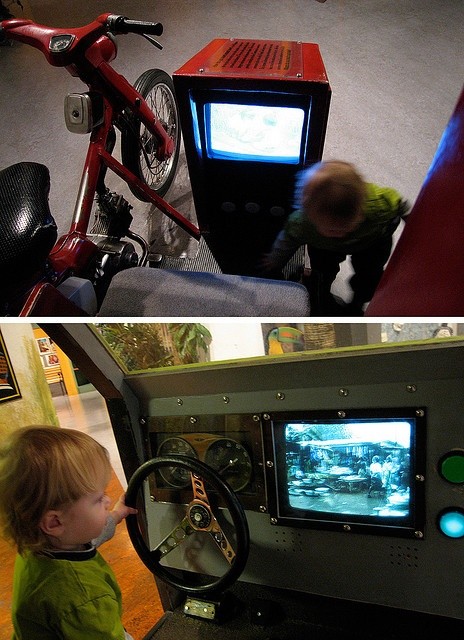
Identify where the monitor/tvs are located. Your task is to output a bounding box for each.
[260,405,429,542]
[195,89,312,170]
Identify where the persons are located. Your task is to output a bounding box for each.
[264,161,414,317]
[0,425,139,640]
[398,454,410,494]
[368,456,382,498]
[381,455,393,496]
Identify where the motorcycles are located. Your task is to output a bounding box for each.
[0,11,201,317]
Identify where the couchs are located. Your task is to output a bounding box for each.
[19,267,309,318]
